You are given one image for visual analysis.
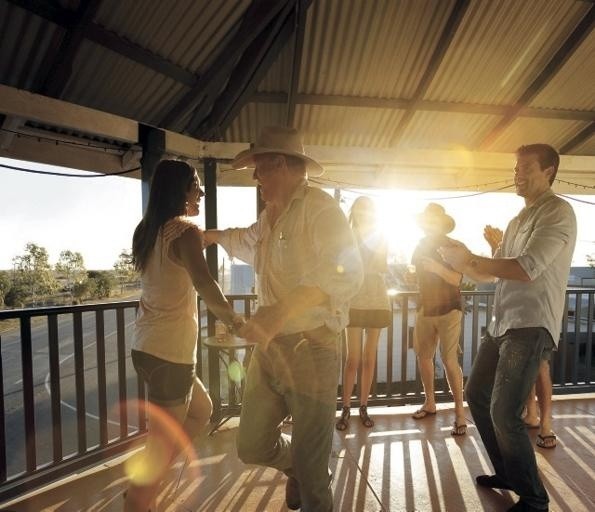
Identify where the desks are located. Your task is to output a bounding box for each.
[204,334,256,437]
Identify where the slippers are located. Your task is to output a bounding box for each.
[411,407,437,419]
[450,420,468,435]
[521,419,540,429]
[535,430,557,448]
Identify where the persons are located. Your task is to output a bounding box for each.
[121,159,253,511]
[197,123,359,512]
[482,222,559,450]
[395,202,469,436]
[437,143,581,511]
[330,194,392,432]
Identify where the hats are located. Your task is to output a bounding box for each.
[230,125,326,179]
[420,203,456,236]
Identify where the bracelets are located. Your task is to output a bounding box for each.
[227,314,248,334]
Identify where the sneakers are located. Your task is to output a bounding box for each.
[504,499,549,511]
[475,474,513,490]
[285,474,302,511]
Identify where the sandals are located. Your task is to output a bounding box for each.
[335,405,351,430]
[358,405,374,428]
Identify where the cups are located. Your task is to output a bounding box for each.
[214,319,226,343]
